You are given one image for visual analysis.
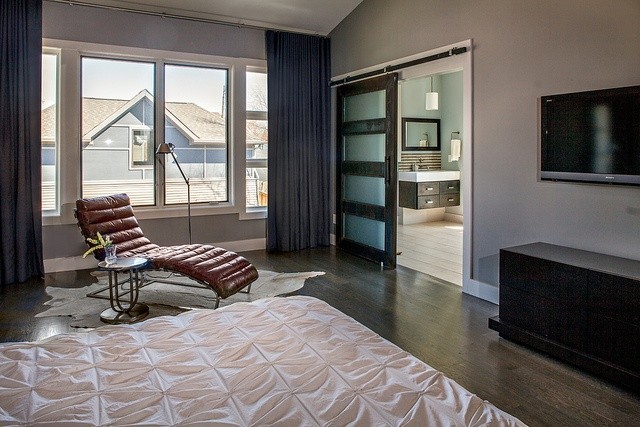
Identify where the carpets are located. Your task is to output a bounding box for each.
[34,265,327,329]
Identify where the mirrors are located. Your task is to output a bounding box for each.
[401,117,442,151]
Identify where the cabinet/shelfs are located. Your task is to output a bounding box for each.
[399,181,460,209]
[481,241,640,394]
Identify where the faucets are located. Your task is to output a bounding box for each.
[419,165,430,170]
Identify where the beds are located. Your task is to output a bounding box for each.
[0,286,533,427]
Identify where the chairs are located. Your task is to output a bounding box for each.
[74,193,258,311]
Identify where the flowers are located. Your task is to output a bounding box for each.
[81,232,114,258]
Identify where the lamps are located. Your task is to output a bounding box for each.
[156,142,192,243]
[425,92,439,111]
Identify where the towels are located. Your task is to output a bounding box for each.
[451,139,461,162]
[419,140,428,147]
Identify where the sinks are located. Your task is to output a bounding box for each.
[418,168,435,171]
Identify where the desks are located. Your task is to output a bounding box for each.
[96,258,149,324]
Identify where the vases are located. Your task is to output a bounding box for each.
[104,245,118,264]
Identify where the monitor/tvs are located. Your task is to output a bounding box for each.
[537,83,640,185]
